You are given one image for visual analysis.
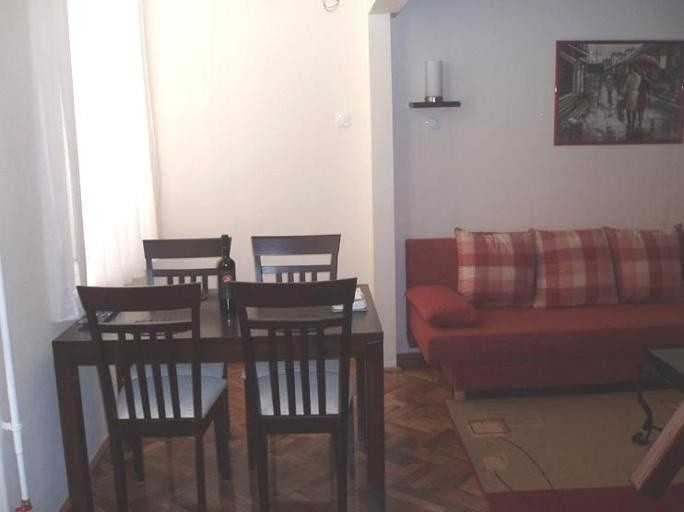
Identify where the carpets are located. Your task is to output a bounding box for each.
[445,387,684,511]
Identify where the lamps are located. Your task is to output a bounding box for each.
[406,58,462,108]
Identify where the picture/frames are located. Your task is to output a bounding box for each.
[555,38,684,144]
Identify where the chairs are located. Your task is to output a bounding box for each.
[240,235,344,377]
[228,278,359,508]
[77,284,229,509]
[115,237,232,392]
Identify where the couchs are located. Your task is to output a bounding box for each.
[404,228,682,400]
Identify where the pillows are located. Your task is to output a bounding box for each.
[454,227,679,310]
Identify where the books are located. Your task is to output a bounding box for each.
[76,308,121,329]
[329,285,369,315]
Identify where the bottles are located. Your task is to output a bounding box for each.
[217,234,236,321]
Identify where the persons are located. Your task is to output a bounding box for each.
[620,60,641,135]
[637,67,652,129]
[606,75,615,109]
